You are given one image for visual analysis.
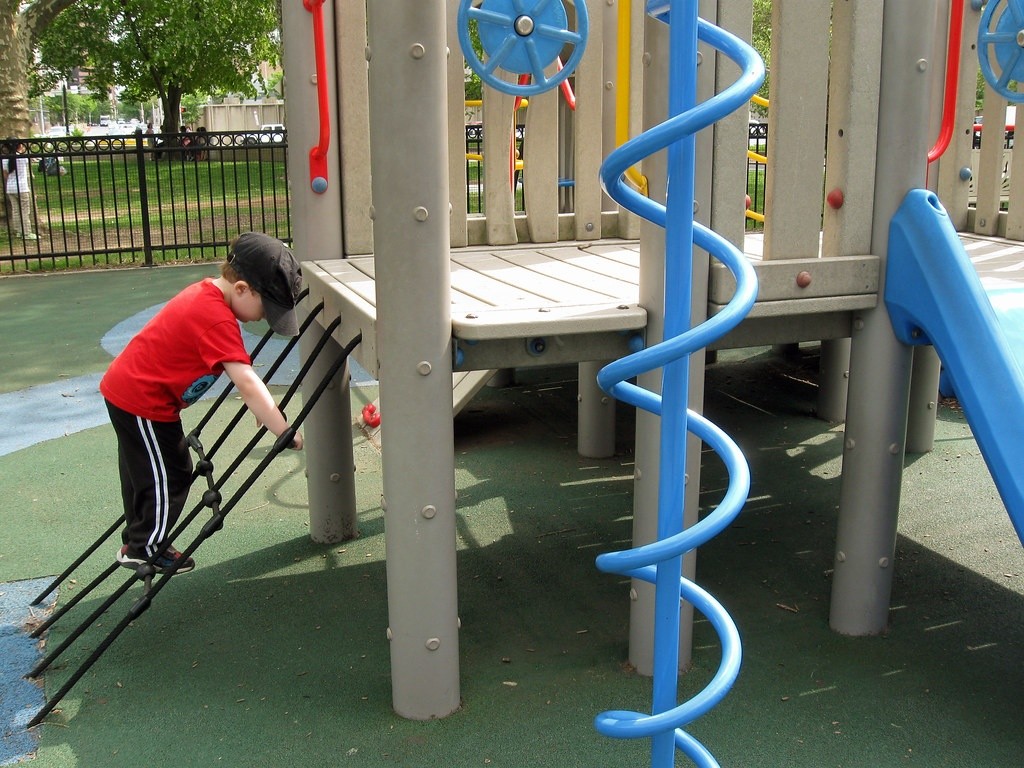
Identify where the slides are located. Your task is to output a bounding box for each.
[885,188,1024,546]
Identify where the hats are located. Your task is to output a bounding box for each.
[227,232,303,336]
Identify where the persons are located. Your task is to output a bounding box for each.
[100,232,304,577]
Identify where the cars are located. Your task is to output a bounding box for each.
[89,114,151,138]
[242,124,286,143]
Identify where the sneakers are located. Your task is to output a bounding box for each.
[24,233,41,239]
[16,233,22,237]
[121,542,195,575]
[117,545,128,561]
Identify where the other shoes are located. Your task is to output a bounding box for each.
[150,158,155,161]
[185,156,209,162]
